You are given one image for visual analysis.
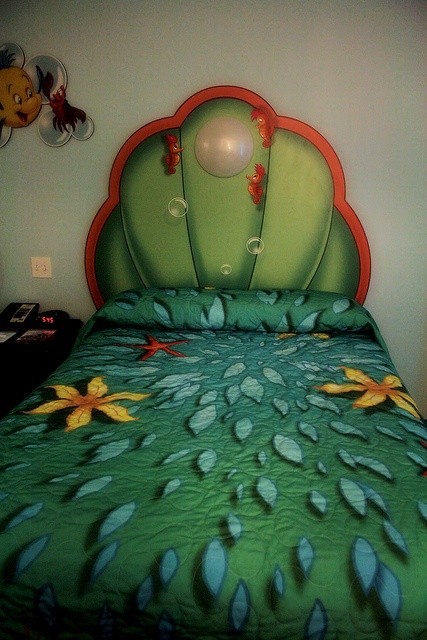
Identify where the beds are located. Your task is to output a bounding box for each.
[0,85,426,636]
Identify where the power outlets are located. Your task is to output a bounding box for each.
[31,256,53,279]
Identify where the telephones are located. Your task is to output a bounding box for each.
[0,302,39,332]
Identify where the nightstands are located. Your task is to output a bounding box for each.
[0,303,79,370]
